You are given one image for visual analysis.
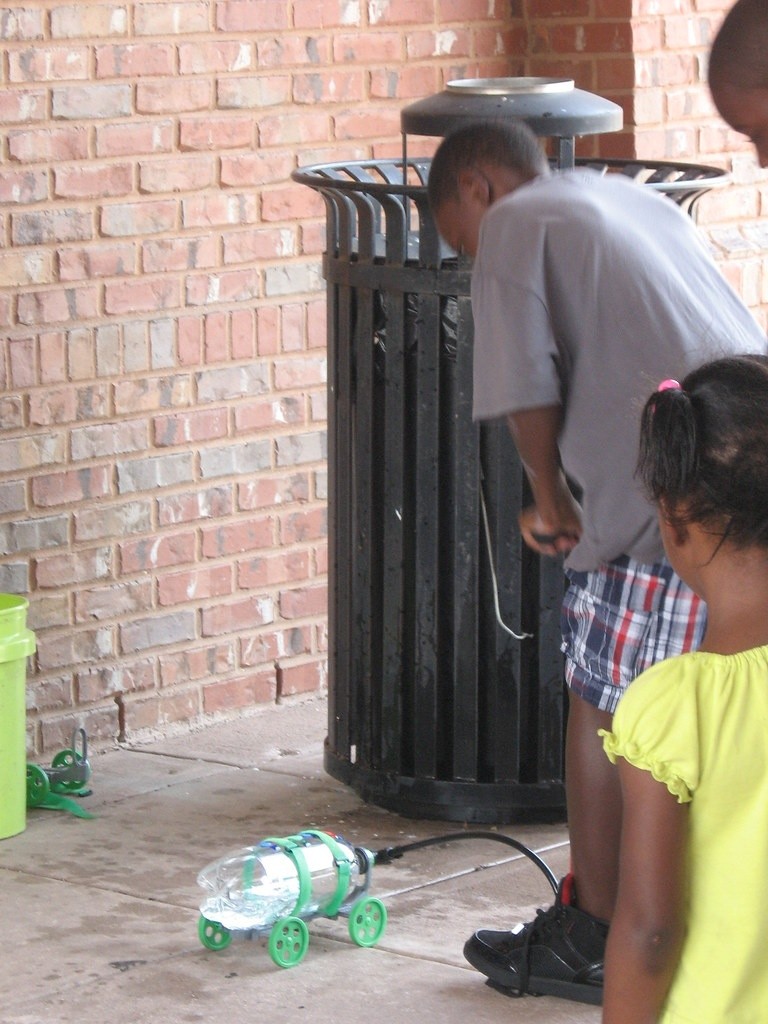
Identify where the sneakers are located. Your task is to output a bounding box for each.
[464,873,610,1005]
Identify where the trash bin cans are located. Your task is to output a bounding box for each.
[290,75,734,826]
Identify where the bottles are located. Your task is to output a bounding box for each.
[195,830,359,932]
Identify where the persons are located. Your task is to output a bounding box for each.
[427,119,768,1024]
[706,0,768,169]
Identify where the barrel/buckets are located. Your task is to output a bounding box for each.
[0,593,37,841]
